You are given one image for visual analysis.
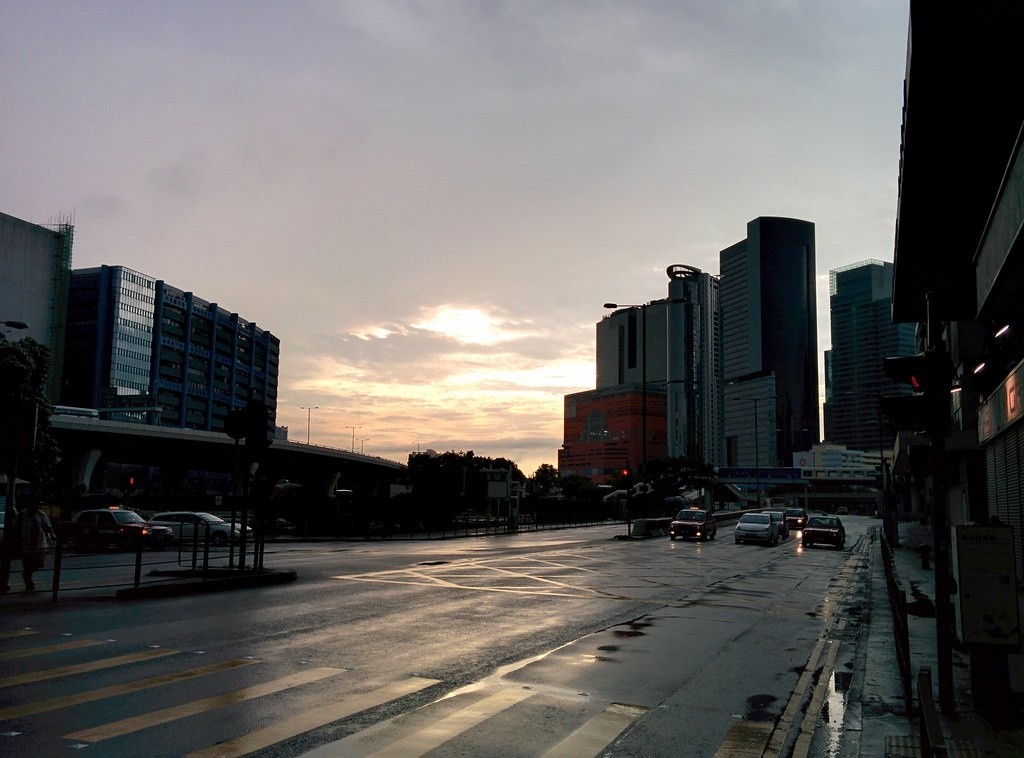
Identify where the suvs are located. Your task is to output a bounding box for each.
[68,508,174,553]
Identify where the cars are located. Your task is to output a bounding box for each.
[760,510,790,540]
[146,512,253,548]
[801,514,846,549]
[669,507,718,542]
[733,513,778,547]
[783,508,809,530]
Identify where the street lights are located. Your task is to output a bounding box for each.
[346,426,361,452]
[731,397,760,509]
[603,303,647,462]
[301,406,318,445]
[355,438,370,454]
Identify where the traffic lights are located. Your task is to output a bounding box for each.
[880,356,930,428]
[621,468,628,476]
